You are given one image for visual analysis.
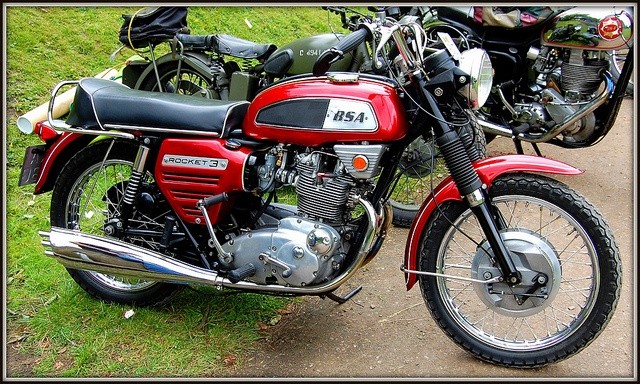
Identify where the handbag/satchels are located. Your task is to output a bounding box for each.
[118,6,188,48]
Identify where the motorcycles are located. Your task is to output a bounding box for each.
[18,11,622,370]
[603,48,636,100]
[93,7,559,229]
